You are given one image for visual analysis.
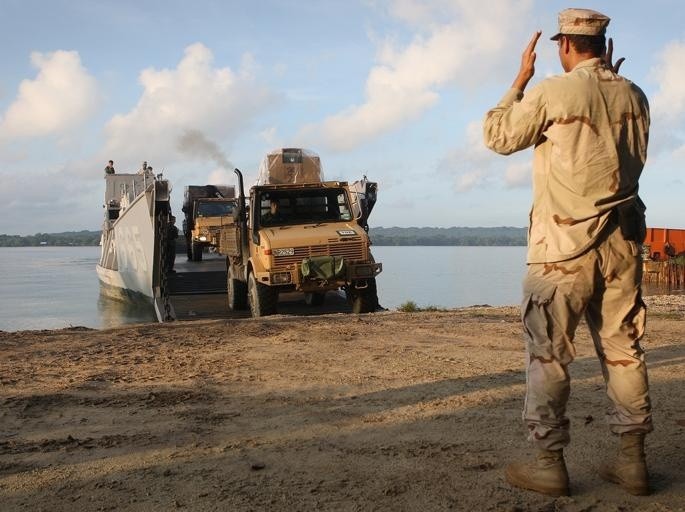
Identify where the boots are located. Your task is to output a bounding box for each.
[598,431,650,495]
[505,446,569,495]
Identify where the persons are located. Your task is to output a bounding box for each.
[104,160,114,175]
[482,6,652,497]
[147,167,155,176]
[136,161,151,175]
[260,199,284,224]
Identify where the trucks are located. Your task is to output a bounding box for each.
[217,168,383,319]
[182,197,251,263]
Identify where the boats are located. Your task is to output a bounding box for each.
[95,169,390,324]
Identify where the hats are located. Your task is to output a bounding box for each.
[550,8,610,40]
